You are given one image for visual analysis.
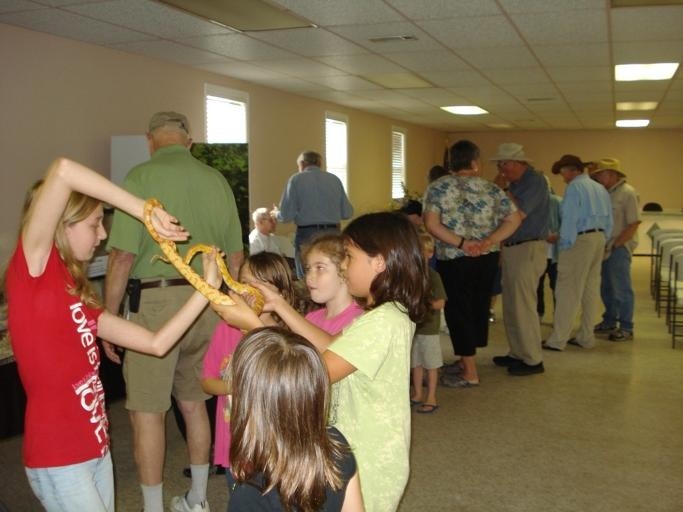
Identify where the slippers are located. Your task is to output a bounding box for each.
[442,373,478,386]
[438,363,462,377]
[419,401,440,413]
[409,396,420,404]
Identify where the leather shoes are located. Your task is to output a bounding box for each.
[568,337,580,345]
[542,339,561,351]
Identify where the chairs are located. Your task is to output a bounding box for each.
[652,229,683,349]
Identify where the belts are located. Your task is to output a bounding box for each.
[294,224,338,231]
[578,228,602,235]
[505,239,535,247]
[142,277,194,288]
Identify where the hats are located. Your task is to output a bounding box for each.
[552,155,593,173]
[148,111,192,138]
[589,157,627,179]
[490,143,534,164]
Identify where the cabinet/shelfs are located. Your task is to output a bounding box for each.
[633,208,683,256]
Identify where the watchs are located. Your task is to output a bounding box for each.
[458,235,464,248]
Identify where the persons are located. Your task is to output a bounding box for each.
[248,208,287,257]
[424,140,521,388]
[2,158,227,512]
[106,111,243,512]
[424,166,449,183]
[537,175,563,323]
[256,233,369,334]
[203,250,296,500]
[592,157,641,342]
[223,328,365,512]
[410,234,447,414]
[487,143,553,374]
[211,212,427,512]
[543,154,613,351]
[271,152,356,284]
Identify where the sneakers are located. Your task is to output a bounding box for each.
[170,492,210,512]
[492,355,511,365]
[506,359,545,375]
[610,327,633,341]
[594,320,617,330]
